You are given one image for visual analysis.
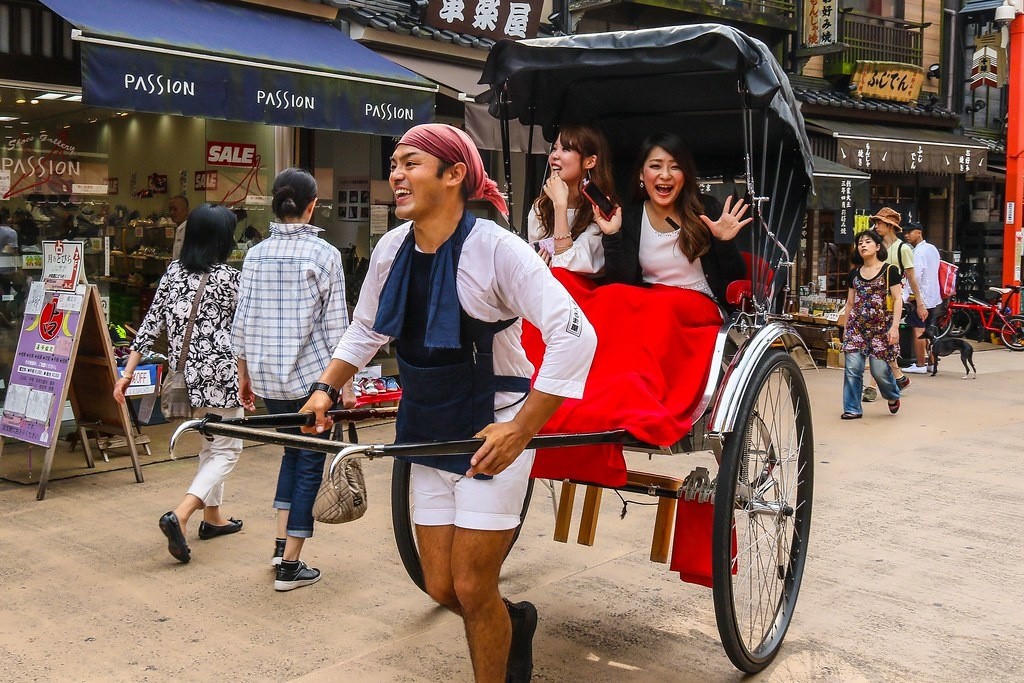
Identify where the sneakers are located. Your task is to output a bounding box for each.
[862,386,877,402]
[897,377,911,392]
[271,539,287,567]
[338,377,399,397]
[901,363,928,373]
[274,560,322,591]
[925,363,934,372]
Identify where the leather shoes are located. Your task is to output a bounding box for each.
[503,597,538,683]
[199,517,243,540]
[159,511,191,563]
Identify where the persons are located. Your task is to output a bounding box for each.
[592,132,753,324]
[839,231,903,420]
[523,126,619,283]
[861,207,929,402]
[242,217,269,261]
[299,122,599,682]
[114,203,245,564]
[15,211,42,289]
[902,222,942,373]
[169,196,190,262]
[0,208,26,328]
[233,167,357,591]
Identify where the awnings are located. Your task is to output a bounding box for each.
[42,1,438,138]
[376,48,552,155]
[805,119,989,175]
[697,148,871,215]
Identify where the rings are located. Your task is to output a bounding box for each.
[553,175,559,179]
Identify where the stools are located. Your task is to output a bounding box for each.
[553,468,679,567]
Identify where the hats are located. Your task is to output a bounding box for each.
[902,222,924,231]
[869,207,903,233]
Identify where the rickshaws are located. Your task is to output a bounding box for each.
[164,18,818,675]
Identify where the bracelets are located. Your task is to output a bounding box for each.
[553,242,573,255]
[553,232,572,240]
[307,381,339,407]
[122,375,132,380]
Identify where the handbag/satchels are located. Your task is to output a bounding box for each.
[885,264,901,312]
[160,370,192,422]
[938,260,959,300]
[312,399,369,524]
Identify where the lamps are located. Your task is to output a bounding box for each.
[965,100,986,114]
[927,63,941,80]
[925,94,940,112]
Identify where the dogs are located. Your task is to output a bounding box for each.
[917,325,977,380]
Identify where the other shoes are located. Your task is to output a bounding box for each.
[888,399,900,414]
[841,412,862,419]
[1,312,17,328]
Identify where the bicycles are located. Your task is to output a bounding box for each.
[925,263,1024,351]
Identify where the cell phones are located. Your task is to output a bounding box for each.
[581,181,616,222]
